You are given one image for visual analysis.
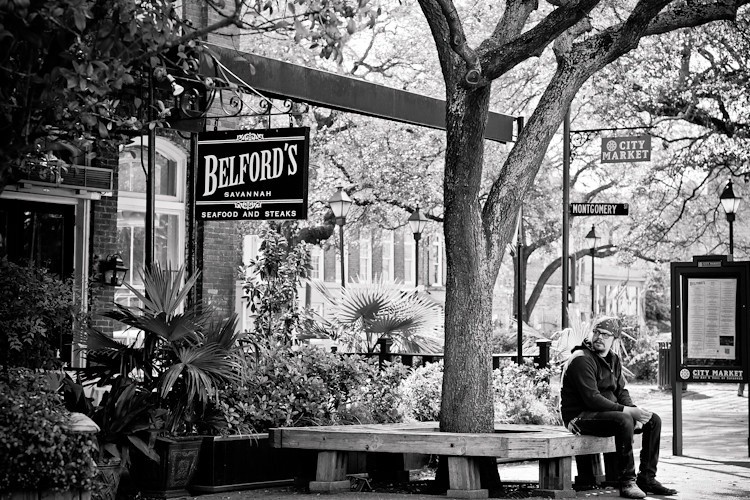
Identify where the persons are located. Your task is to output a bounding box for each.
[560,317,678,498]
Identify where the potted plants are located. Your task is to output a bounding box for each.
[68,258,242,499]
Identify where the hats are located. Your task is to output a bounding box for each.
[593,318,622,338]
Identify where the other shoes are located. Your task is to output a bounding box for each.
[738,390,743,396]
[636,474,677,495]
[620,477,646,498]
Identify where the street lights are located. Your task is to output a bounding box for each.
[408,202,428,288]
[328,186,353,289]
[719,178,743,262]
[585,224,602,320]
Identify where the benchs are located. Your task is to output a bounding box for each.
[268,421,615,500]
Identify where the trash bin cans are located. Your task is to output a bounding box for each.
[658,341,687,390]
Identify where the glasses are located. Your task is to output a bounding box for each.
[593,329,614,339]
